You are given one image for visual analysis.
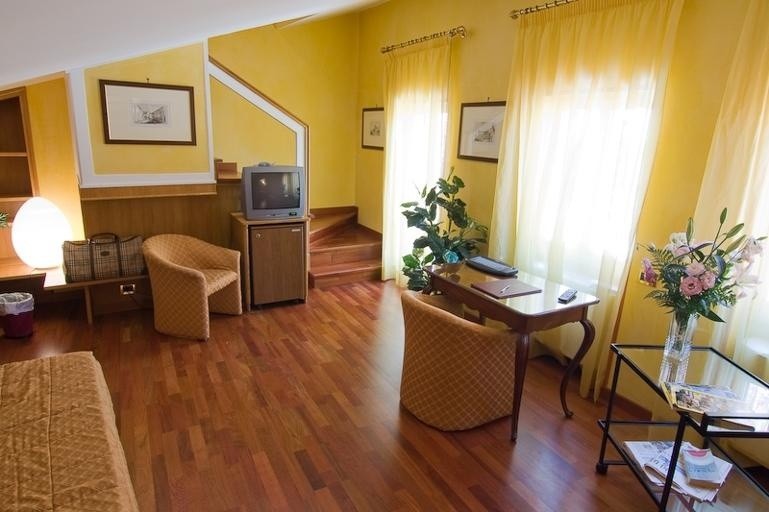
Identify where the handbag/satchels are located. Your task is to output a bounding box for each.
[63,232,145,282]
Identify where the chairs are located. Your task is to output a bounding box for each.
[142,234,243,342]
[398,289,518,434]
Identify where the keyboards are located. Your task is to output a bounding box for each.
[465,255,518,277]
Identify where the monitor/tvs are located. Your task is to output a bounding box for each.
[240,165,305,221]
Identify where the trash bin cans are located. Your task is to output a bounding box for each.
[0,292,35,338]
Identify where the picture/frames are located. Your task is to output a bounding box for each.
[362,107,385,150]
[457,100,507,163]
[98,79,197,146]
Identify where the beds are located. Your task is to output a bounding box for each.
[0,350,139,511]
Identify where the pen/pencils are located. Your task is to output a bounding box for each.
[501,285,510,293]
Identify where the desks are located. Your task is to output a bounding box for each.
[422,261,600,443]
[43,270,149,326]
[231,212,309,312]
[595,342,769,512]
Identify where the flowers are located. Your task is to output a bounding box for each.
[637,207,767,350]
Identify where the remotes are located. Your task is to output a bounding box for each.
[558,289,577,302]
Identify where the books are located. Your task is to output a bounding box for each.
[682,447,721,487]
[659,378,754,415]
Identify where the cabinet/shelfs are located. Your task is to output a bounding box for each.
[0,85,46,282]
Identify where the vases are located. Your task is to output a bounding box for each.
[658,308,698,385]
